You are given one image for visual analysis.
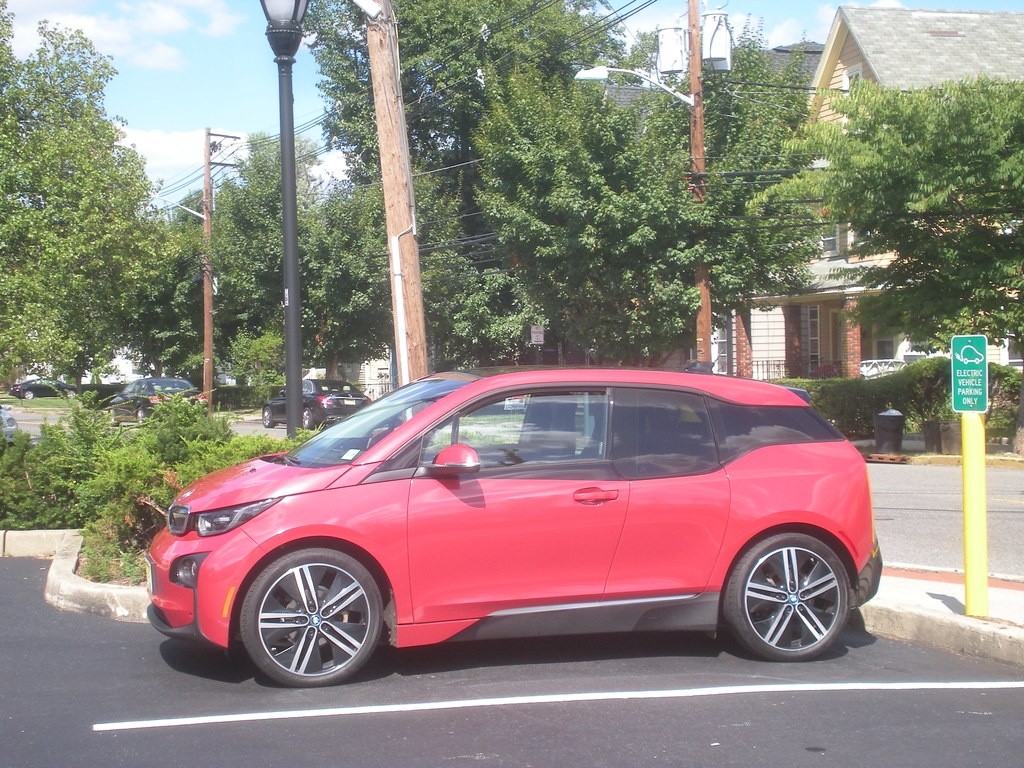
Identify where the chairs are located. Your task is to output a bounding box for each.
[518,392,577,455]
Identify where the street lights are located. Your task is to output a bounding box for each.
[257,0,312,443]
[572,64,713,375]
[144,191,213,414]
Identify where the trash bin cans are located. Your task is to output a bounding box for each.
[923,422,941,453]
[873,415,904,454]
[939,420,962,455]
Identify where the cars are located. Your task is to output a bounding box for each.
[8,376,78,400]
[260,378,374,431]
[0,405,20,448]
[108,376,209,427]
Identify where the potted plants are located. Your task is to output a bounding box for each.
[932,408,962,455]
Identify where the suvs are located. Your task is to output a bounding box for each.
[147,362,884,690]
